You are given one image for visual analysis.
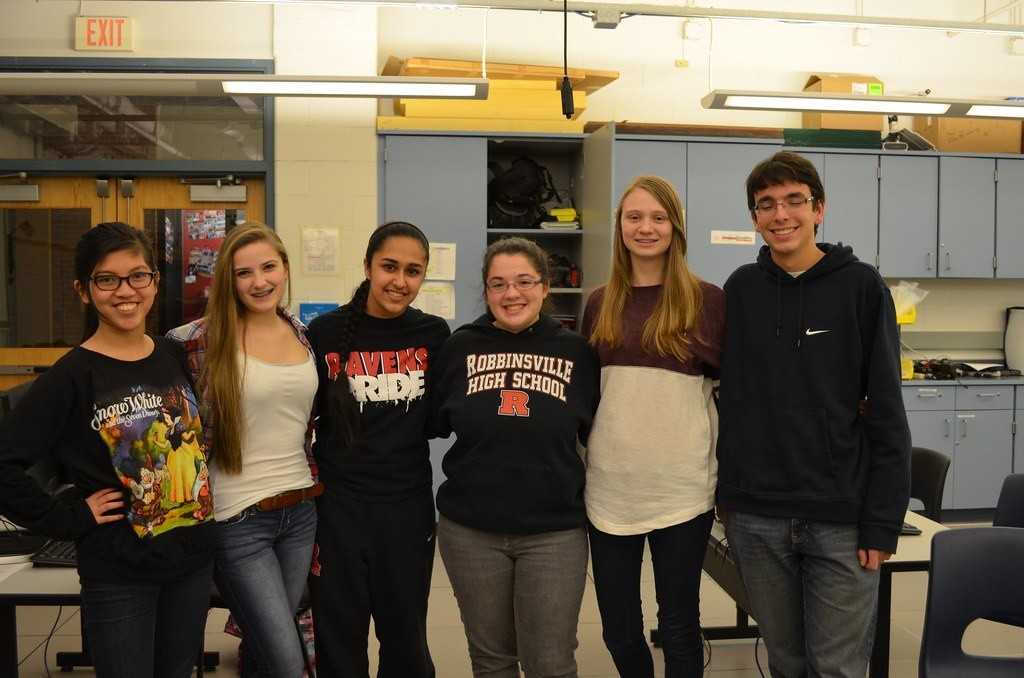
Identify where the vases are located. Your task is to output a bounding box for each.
[1004,307,1024,376]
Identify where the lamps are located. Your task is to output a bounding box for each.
[1,5,489,100]
[702,13,1024,117]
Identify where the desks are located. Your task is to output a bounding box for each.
[651,509,949,677]
[0,562,220,678]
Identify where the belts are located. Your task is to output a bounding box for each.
[256,483,325,513]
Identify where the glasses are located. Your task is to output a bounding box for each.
[88,270,157,291]
[486,278,543,294]
[753,196,814,217]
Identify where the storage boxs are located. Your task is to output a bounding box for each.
[913,115,1024,155]
[802,73,884,130]
[374,57,620,132]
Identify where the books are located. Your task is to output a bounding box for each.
[540,222,580,230]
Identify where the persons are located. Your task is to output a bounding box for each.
[716,152,911,678]
[0,222,217,678]
[303,222,452,678]
[579,175,866,678]
[426,237,600,678]
[164,223,319,678]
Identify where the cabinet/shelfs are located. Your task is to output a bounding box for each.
[900,384,1023,509]
[380,122,616,523]
[615,138,1024,279]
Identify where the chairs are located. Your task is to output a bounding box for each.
[918,527,1024,678]
[992,473,1024,528]
[911,447,951,524]
